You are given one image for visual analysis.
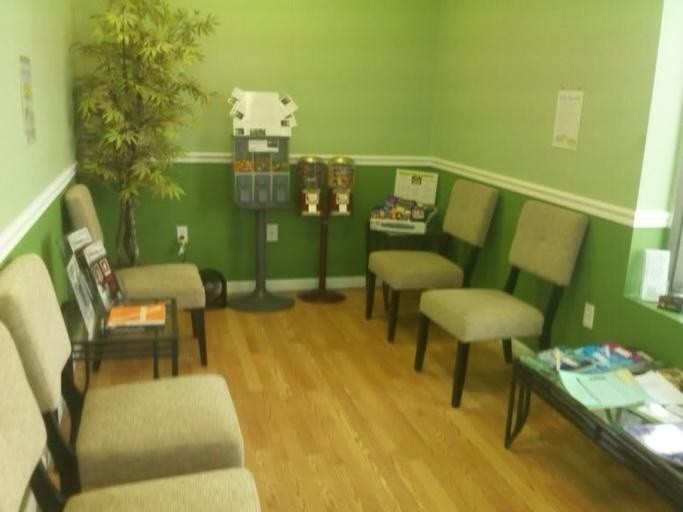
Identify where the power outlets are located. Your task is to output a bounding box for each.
[176,226,188,244]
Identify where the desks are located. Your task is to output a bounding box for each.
[503,341,683,510]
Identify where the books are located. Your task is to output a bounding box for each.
[537,342,658,373]
[622,423,683,467]
[556,369,646,410]
[630,366,683,422]
[106,303,166,329]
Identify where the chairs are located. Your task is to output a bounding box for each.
[363,178,496,342]
[0,252,246,497]
[413,199,590,408]
[0,322,262,512]
[63,183,208,367]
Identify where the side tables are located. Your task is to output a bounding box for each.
[58,296,179,379]
